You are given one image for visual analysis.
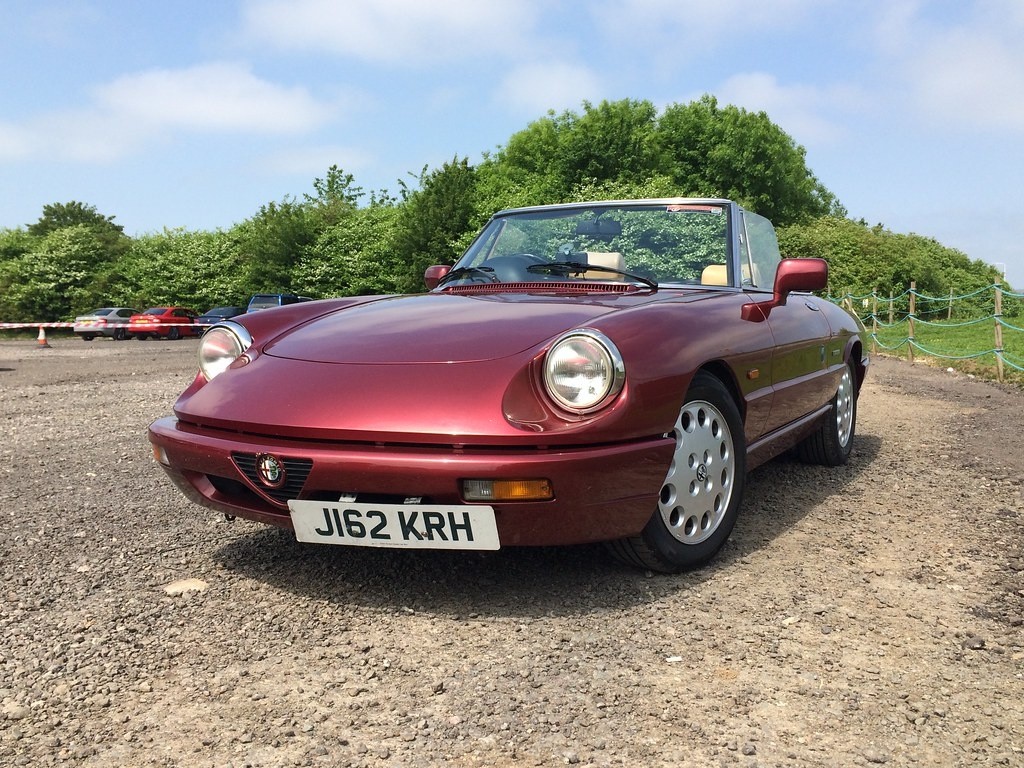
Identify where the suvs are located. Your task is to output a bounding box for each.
[247,295,313,314]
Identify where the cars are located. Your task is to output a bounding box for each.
[194,307,247,333]
[128,307,201,339]
[73,307,141,340]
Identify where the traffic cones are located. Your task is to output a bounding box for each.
[36,327,50,349]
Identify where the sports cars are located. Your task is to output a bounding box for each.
[150,197,870,572]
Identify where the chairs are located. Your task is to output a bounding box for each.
[701,263,761,287]
[568,252,626,282]
